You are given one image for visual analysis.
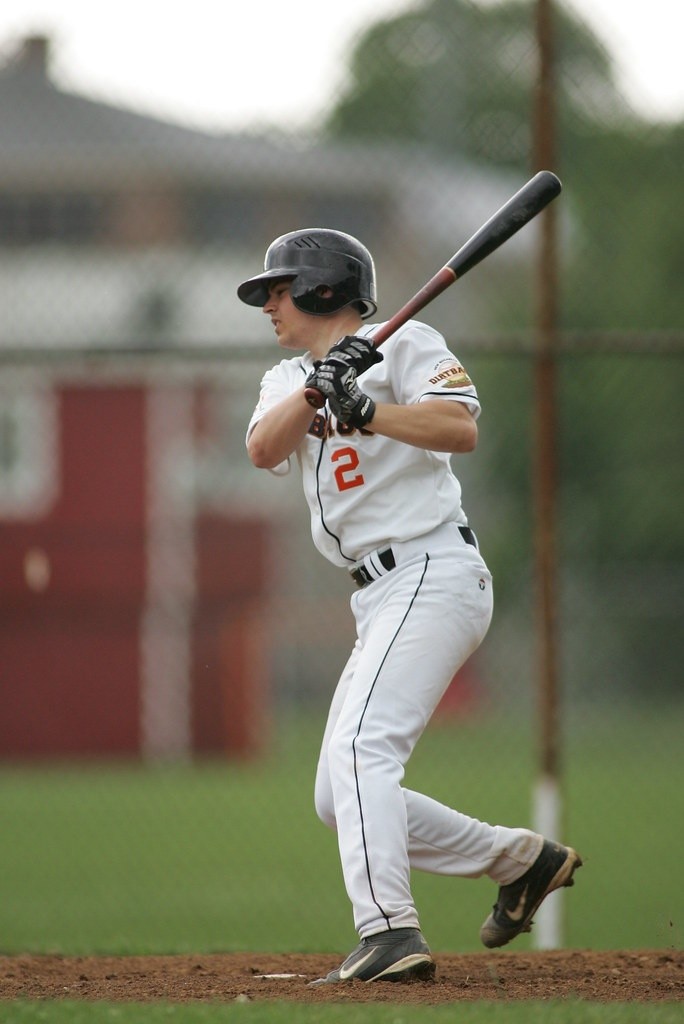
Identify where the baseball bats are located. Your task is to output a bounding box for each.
[302,168,565,409]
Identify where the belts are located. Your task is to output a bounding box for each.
[350,527,476,588]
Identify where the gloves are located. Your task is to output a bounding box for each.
[305,335,383,394]
[313,357,375,429]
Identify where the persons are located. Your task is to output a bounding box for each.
[238,228,582,985]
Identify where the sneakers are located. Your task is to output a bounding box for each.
[308,928,436,984]
[481,838,583,948]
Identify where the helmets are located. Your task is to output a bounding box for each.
[237,228,377,319]
